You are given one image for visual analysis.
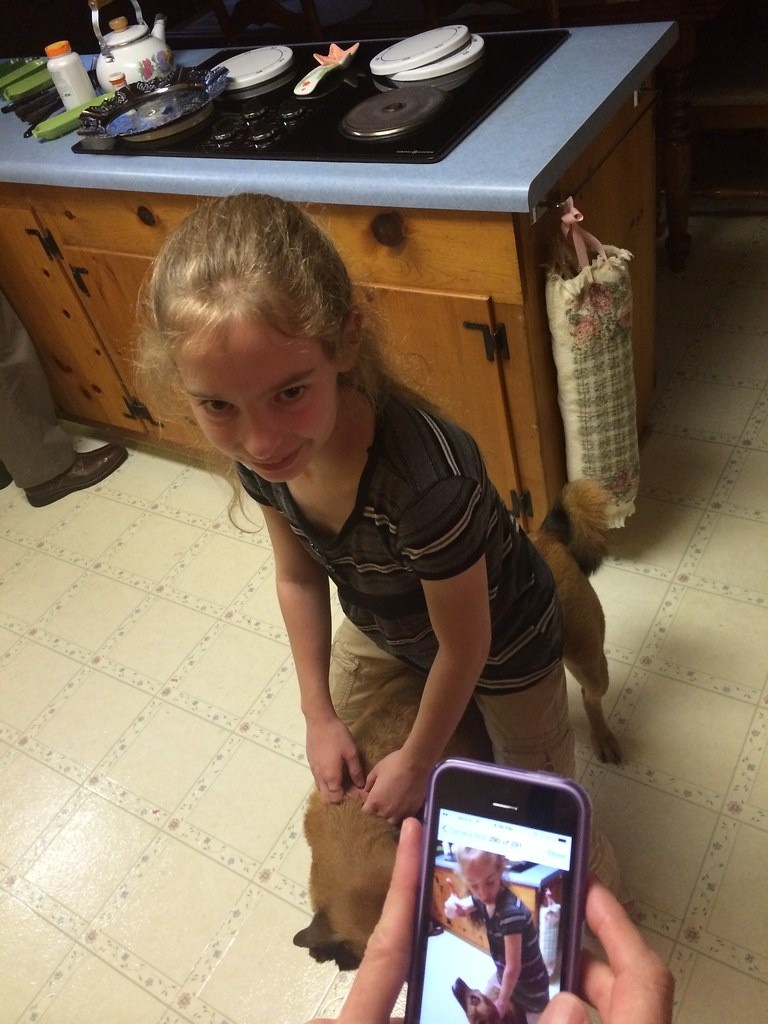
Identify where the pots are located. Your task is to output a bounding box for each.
[78,66,233,142]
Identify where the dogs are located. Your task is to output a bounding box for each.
[451,977,526,1024]
[294,476,621,972]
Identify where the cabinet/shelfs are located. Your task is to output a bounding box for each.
[0,72,663,544]
[430,866,564,957]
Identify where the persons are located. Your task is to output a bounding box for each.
[443,848,548,1016]
[0,289,129,507]
[303,815,674,1024]
[137,193,631,906]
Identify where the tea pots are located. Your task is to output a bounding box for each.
[89,0,173,92]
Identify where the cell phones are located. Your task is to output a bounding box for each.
[403,756,593,1024]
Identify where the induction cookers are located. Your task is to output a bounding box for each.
[72,29,571,163]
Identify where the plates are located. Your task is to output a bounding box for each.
[0,58,55,101]
[32,90,115,140]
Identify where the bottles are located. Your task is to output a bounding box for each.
[108,72,127,92]
[44,40,97,112]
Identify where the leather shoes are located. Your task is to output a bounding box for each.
[0,459,13,490]
[428,926,444,936]
[23,442,129,507]
